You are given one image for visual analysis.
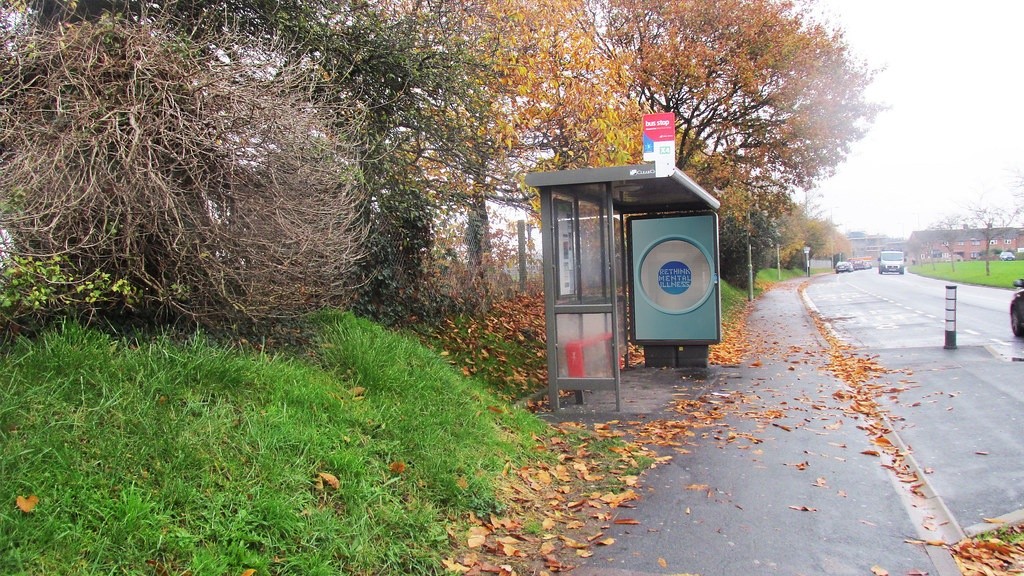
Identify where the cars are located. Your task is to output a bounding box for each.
[836,261,872,274]
[999,252,1016,261]
[1010,279,1024,338]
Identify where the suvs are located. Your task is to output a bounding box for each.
[878,249,907,274]
